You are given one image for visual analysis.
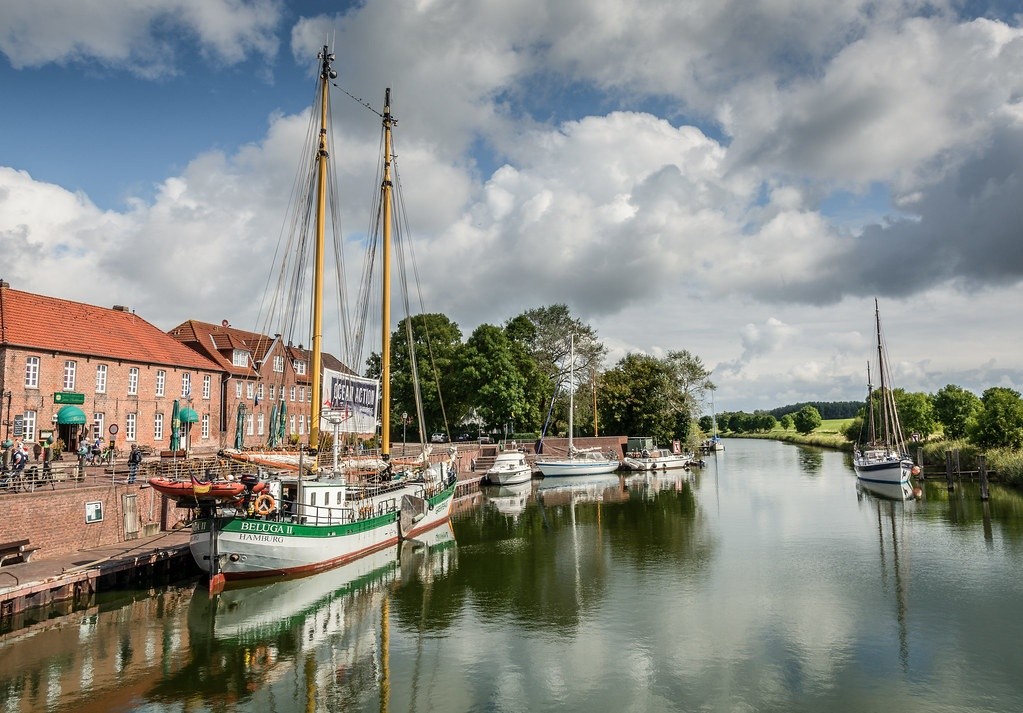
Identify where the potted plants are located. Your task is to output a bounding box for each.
[51,438,67,460]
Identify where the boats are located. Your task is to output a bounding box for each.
[627,449,688,469]
[149,474,270,509]
[691,457,706,466]
[484,449,533,484]
[623,457,646,471]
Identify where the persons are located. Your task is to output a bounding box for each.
[81,438,92,461]
[12,449,25,490]
[511,440,517,449]
[127,444,137,484]
[95,437,102,450]
[358,442,363,451]
[349,443,354,452]
[499,443,503,452]
[3,446,12,475]
[471,458,476,472]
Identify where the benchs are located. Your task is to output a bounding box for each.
[160,451,186,460]
[0,538,41,568]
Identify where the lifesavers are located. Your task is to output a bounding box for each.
[254,494,275,515]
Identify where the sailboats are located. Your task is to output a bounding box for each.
[706,389,724,450]
[857,477,917,670]
[534,334,619,476]
[186,40,473,590]
[851,296,920,485]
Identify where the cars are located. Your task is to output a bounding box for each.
[431,432,450,443]
[477,433,496,444]
[455,433,473,441]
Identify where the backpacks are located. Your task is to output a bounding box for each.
[132,451,142,463]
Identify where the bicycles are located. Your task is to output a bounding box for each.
[0,462,55,494]
[80,448,111,468]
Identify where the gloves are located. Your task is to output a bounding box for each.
[127,460,131,466]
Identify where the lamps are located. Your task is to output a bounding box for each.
[51,414,58,422]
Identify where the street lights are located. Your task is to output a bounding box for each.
[402,411,408,454]
